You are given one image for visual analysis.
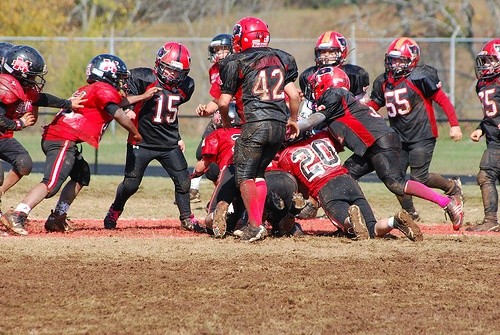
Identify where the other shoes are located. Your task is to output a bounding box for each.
[271,190,285,210]
[290,191,306,210]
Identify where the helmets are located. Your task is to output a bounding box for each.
[476,37,500,79]
[0,45,49,94]
[208,33,234,55]
[212,101,239,129]
[384,37,420,75]
[154,42,192,87]
[314,31,348,66]
[0,42,14,61]
[232,16,271,52]
[84,53,130,94]
[304,66,351,102]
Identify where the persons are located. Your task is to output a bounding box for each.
[470,38,500,233]
[187,16,301,243]
[296,67,463,231]
[0,42,89,231]
[0,54,163,236]
[271,127,424,242]
[103,42,198,229]
[300,31,370,106]
[364,36,463,221]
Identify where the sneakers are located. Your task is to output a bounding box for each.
[232,224,268,242]
[213,200,228,238]
[0,206,31,237]
[104,203,125,229]
[45,209,76,232]
[393,209,423,241]
[443,195,463,231]
[467,219,500,232]
[347,205,370,240]
[408,210,421,222]
[181,218,196,230]
[444,176,465,204]
